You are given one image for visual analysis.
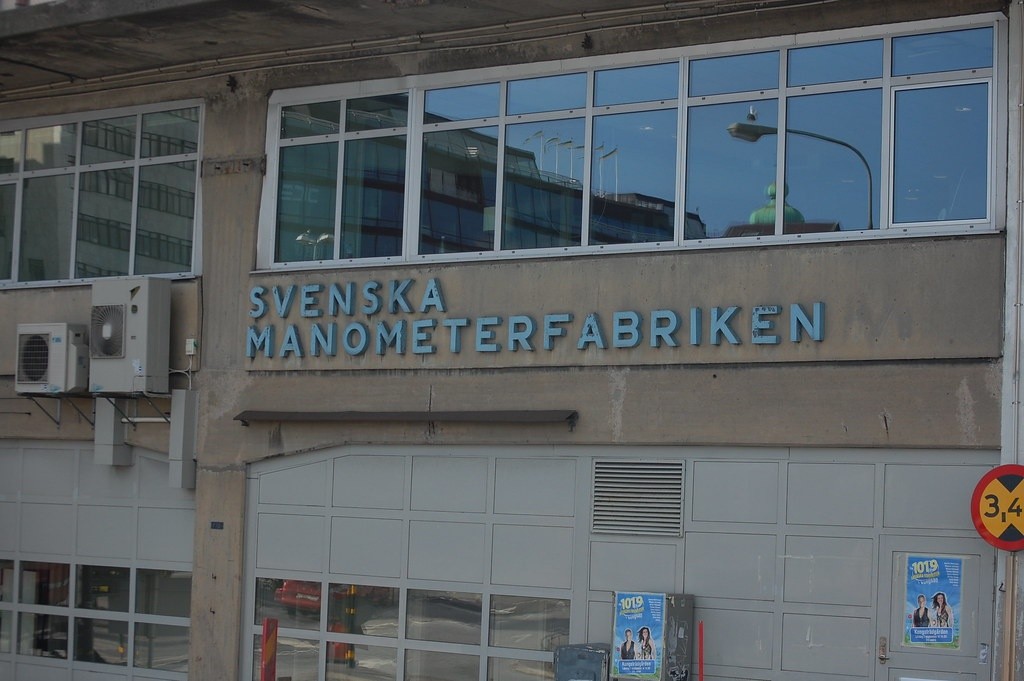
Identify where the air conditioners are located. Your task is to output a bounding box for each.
[88,277,171,394]
[14,322,90,394]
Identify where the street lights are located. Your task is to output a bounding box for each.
[725,122,874,231]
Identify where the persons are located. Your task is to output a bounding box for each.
[912,594,933,627]
[930,592,954,628]
[619,628,638,660]
[637,625,656,659]
